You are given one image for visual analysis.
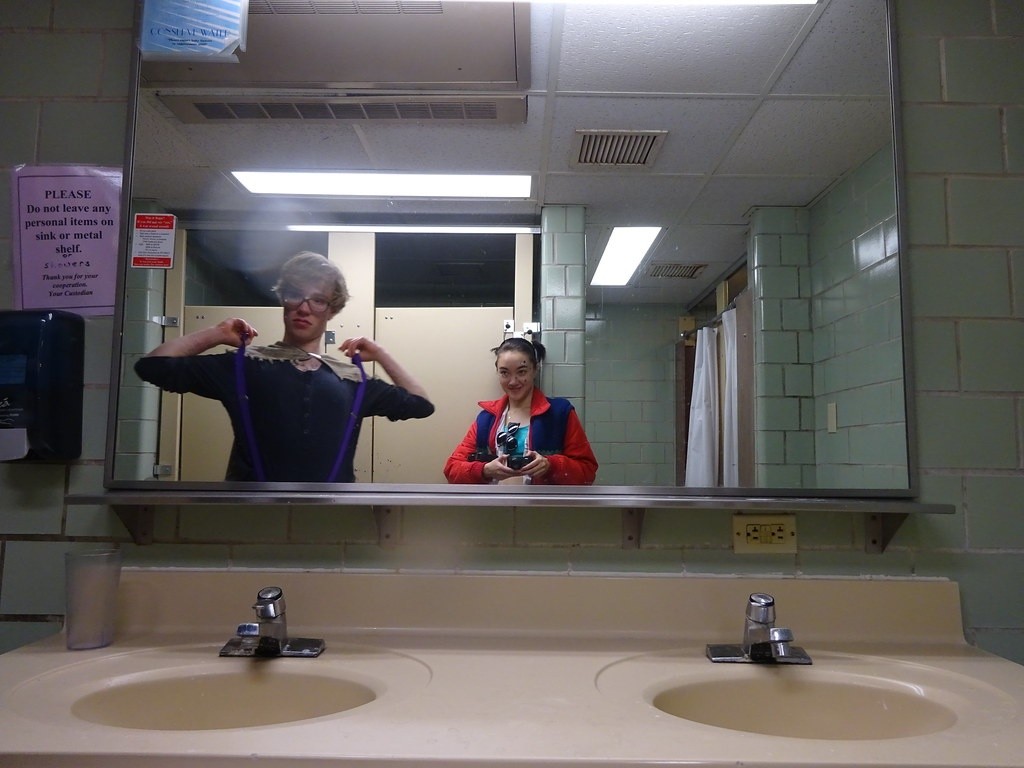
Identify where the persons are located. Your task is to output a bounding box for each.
[443,338,599,486]
[133,252,435,483]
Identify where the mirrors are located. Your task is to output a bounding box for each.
[100,0,922,500]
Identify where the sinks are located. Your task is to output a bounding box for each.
[590,644,1016,751]
[8,642,434,737]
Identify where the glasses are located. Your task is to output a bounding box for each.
[282,289,331,312]
[496,422,520,449]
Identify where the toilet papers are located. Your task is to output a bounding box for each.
[0,427,32,462]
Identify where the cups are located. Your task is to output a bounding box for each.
[64,549,122,650]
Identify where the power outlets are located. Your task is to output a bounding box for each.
[731,513,798,553]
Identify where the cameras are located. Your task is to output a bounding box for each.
[507,455,533,470]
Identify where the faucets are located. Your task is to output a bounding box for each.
[706,590,813,664]
[218,585,326,661]
